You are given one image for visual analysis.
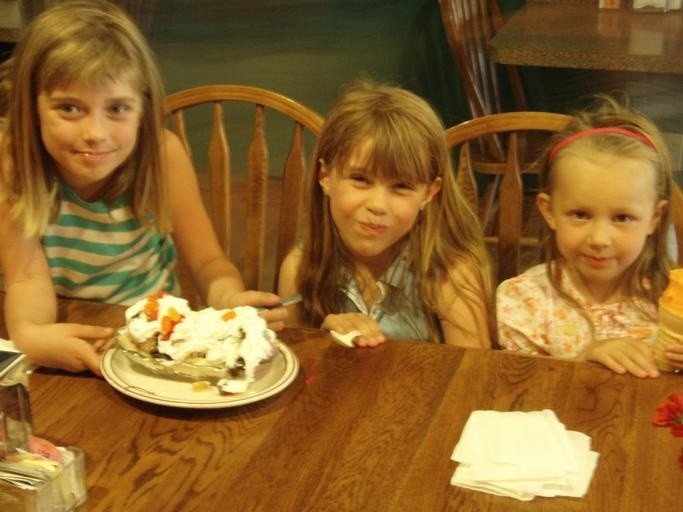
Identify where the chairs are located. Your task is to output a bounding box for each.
[441,111,583,352]
[437,0,551,252]
[160,85,326,326]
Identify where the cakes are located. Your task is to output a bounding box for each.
[116,290,277,395]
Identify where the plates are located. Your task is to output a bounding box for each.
[95,337,303,411]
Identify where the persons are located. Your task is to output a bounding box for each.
[0,2,287,384]
[269,81,494,356]
[493,105,682,383]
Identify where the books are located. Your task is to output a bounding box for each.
[0,435,85,511]
